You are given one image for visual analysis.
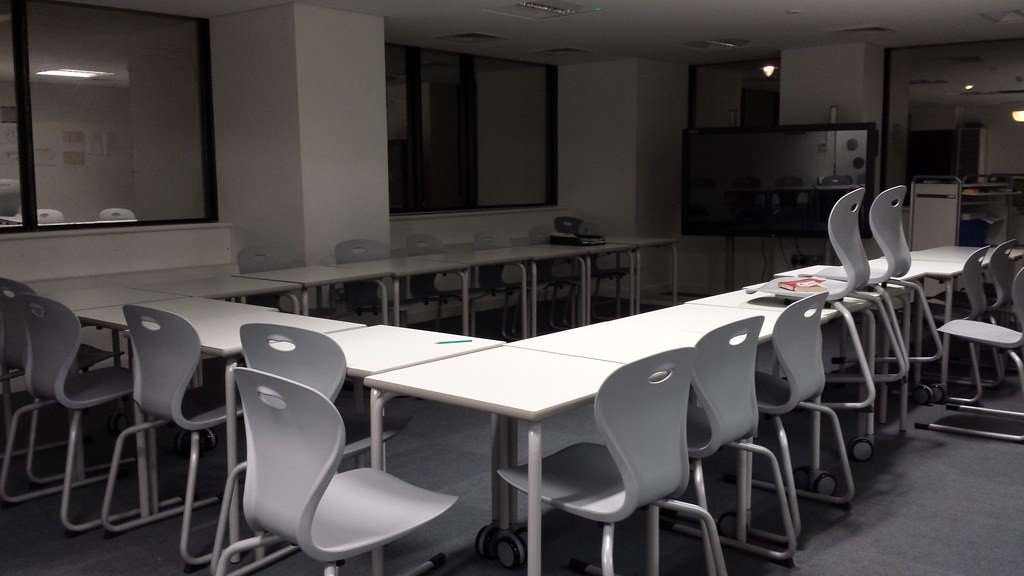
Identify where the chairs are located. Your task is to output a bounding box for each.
[475,184,1023,574]
[0,222,459,576]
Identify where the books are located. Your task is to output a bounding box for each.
[998,188,1017,192]
[963,188,984,192]
[778,277,828,293]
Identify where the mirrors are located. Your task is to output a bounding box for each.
[688,55,780,131]
[877,39,1024,212]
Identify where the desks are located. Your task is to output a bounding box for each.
[907,173,1011,297]
[1006,173,1023,248]
[50,235,1022,575]
